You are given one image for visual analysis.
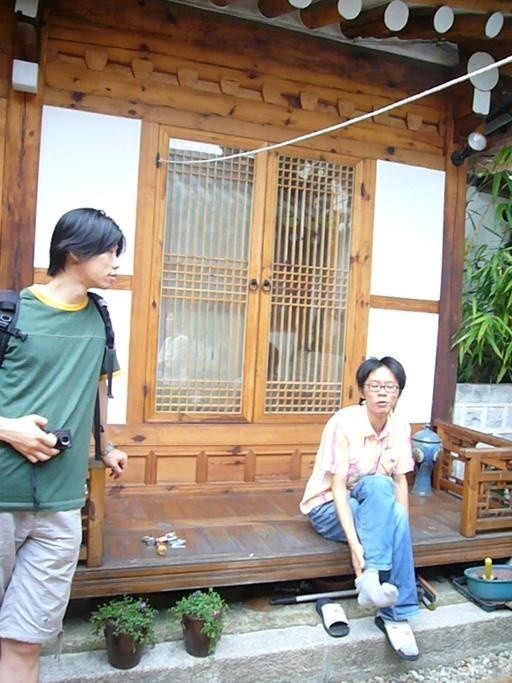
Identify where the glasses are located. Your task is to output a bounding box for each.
[362,383,400,393]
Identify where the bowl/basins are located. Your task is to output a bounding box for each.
[464,565,512,601]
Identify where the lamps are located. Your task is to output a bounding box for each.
[452,126,490,168]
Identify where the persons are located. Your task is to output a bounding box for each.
[0,209,126,683]
[299,356,422,662]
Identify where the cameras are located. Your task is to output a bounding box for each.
[39,429,72,450]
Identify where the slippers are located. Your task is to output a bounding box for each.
[375,615,420,660]
[315,598,349,637]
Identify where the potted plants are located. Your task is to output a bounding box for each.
[169,591,227,658]
[92,598,155,671]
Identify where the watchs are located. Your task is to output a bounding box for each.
[97,442,114,457]
